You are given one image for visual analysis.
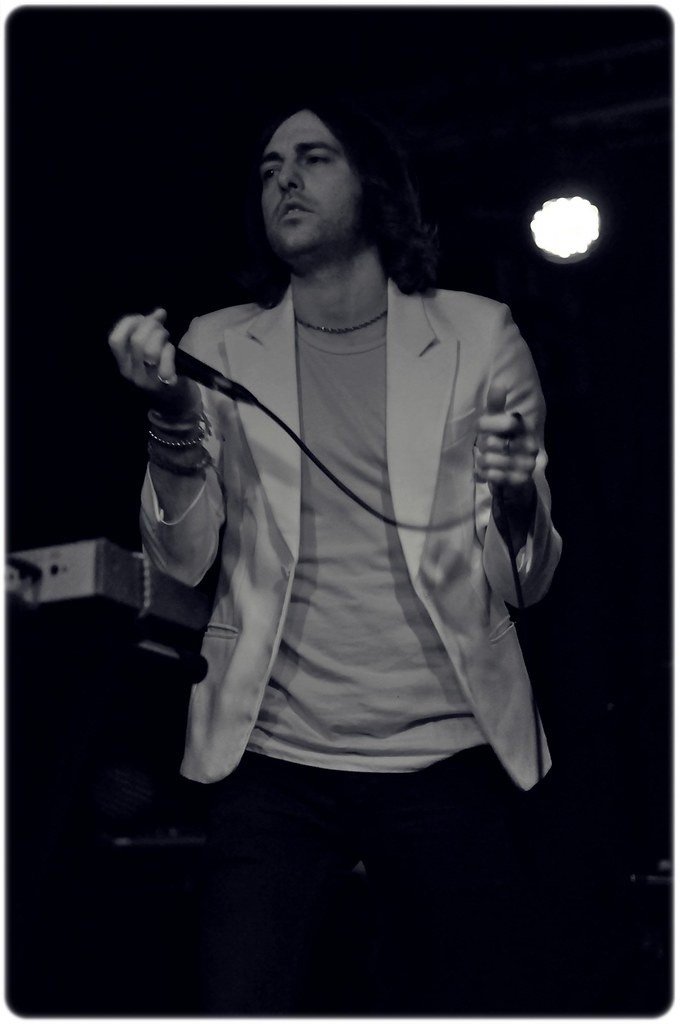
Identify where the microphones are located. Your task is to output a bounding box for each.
[110,317,256,407]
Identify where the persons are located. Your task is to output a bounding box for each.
[106,101,562,1015]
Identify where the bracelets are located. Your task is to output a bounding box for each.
[148,410,201,433]
[146,427,205,450]
[148,442,210,476]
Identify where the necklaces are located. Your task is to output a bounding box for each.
[294,313,389,334]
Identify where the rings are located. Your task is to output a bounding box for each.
[152,374,174,387]
[504,437,510,453]
[143,359,160,369]
